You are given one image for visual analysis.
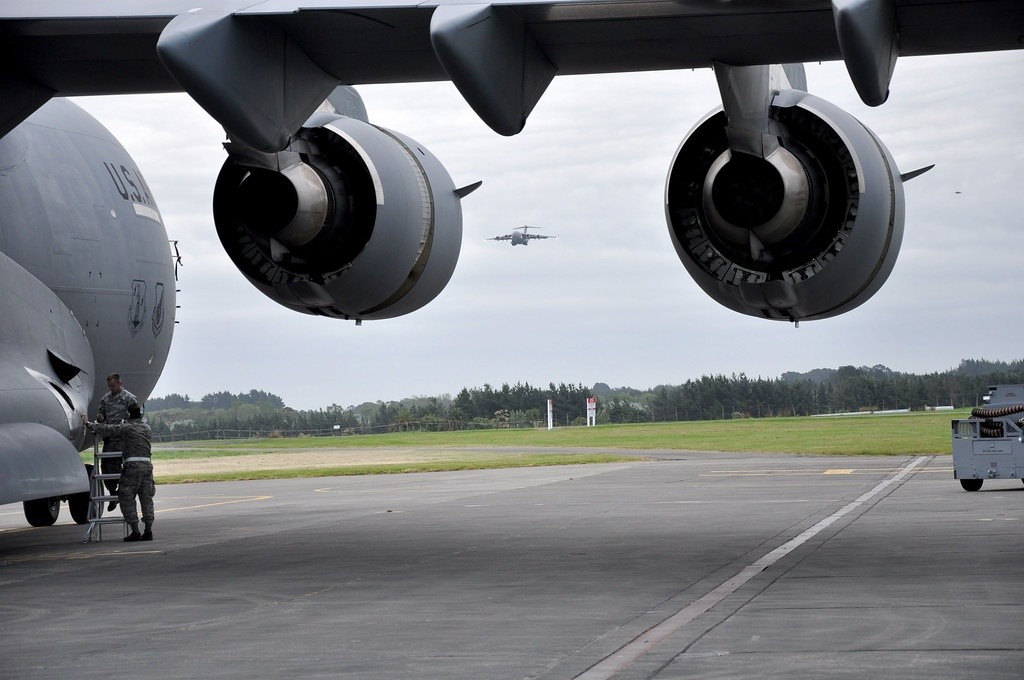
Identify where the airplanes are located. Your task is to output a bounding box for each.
[486,225,558,246]
[0,0,1023,526]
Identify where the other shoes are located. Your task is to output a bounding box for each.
[107,491,119,511]
[141,530,153,541]
[123,532,141,541]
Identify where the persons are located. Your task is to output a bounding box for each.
[80,404,155,542]
[97,373,143,511]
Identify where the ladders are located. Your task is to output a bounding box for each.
[83,424,129,545]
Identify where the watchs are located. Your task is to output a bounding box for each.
[86,422,90,426]
